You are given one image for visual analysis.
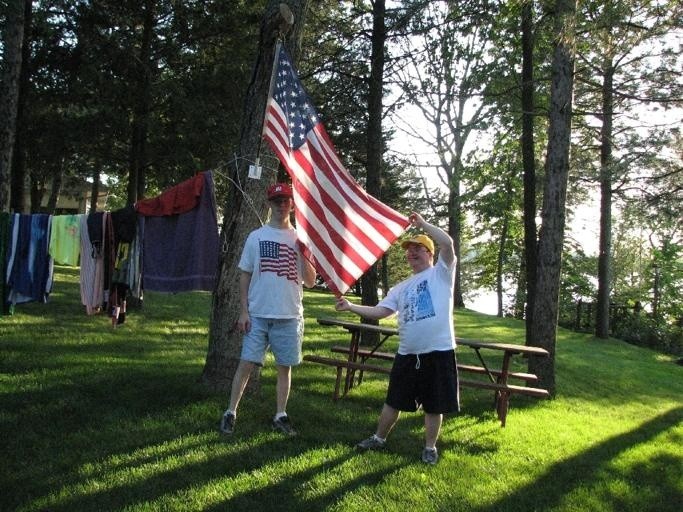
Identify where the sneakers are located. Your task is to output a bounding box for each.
[422,447,437,464]
[222,412,236,434]
[354,435,386,449]
[272,416,297,438]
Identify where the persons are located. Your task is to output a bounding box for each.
[336,211,462,464]
[221,183,316,437]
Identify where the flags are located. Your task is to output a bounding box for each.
[263,41,412,298]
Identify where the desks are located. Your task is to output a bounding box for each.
[315,315,548,397]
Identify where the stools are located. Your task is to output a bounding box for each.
[303,344,542,426]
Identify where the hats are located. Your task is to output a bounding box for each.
[402,234,434,255]
[267,183,293,200]
